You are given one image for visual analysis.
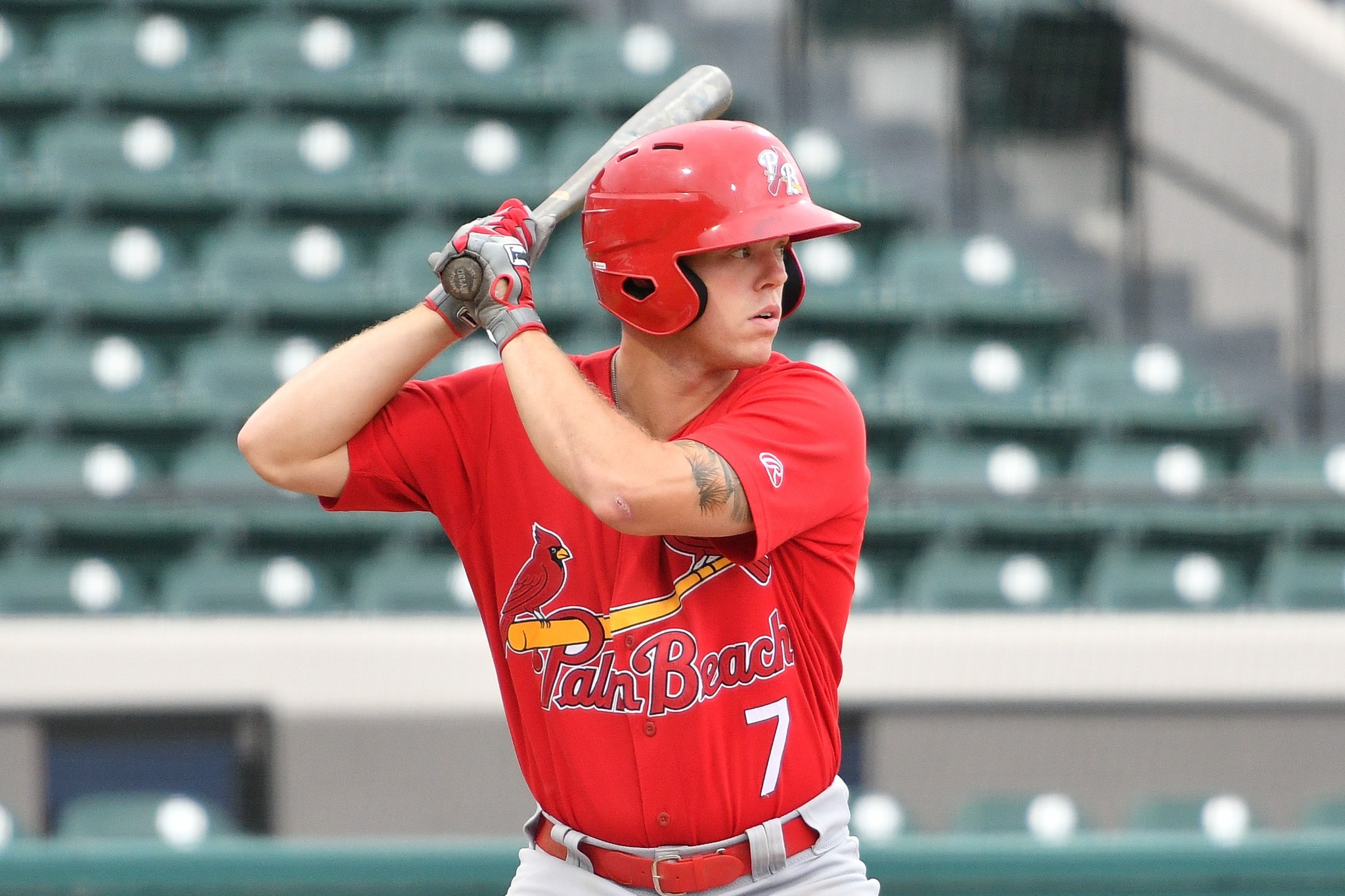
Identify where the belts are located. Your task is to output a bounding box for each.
[535,812,820,896]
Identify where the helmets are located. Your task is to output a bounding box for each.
[582,120,860,337]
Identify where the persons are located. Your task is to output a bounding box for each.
[235,116,882,896]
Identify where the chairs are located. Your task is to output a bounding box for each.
[0,0,1345,895]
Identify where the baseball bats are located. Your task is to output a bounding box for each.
[438,61,735,306]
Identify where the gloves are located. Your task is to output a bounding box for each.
[424,198,547,360]
